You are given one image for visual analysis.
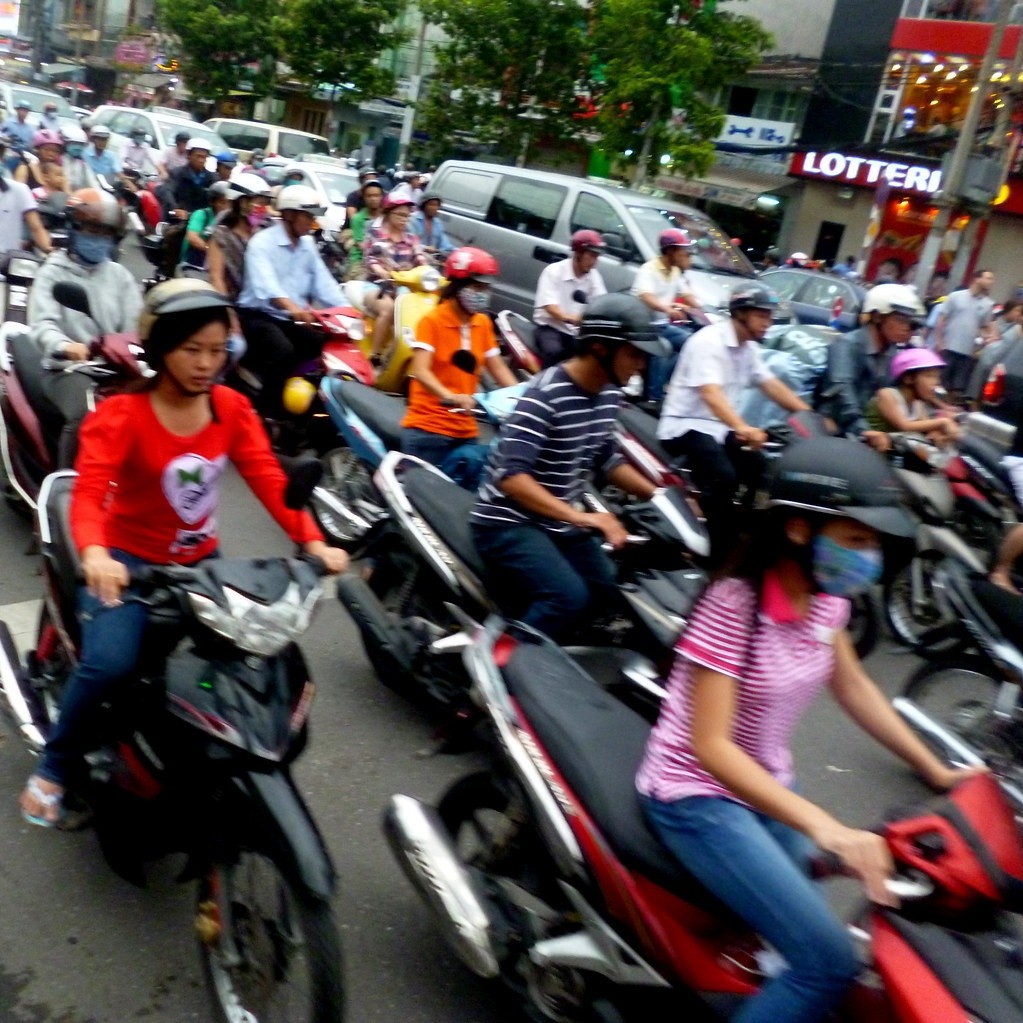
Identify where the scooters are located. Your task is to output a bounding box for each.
[0,142,1023,1022]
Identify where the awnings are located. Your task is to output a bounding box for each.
[644,161,800,209]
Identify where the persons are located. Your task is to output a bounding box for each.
[532,226,1023,1022]
[0,102,672,1023]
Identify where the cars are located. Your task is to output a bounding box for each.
[754,268,868,333]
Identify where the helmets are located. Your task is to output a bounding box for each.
[762,435,921,537]
[1,97,445,217]
[574,292,676,363]
[892,346,948,377]
[363,178,381,189]
[728,281,781,311]
[659,228,696,250]
[569,228,606,255]
[859,282,929,327]
[603,231,631,256]
[67,186,126,232]
[134,275,235,370]
[444,246,500,284]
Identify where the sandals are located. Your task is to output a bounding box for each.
[17,774,70,833]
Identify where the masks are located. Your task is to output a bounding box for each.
[457,288,491,315]
[797,533,885,598]
[70,231,114,265]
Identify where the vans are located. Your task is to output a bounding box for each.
[0,82,362,244]
[423,159,796,335]
